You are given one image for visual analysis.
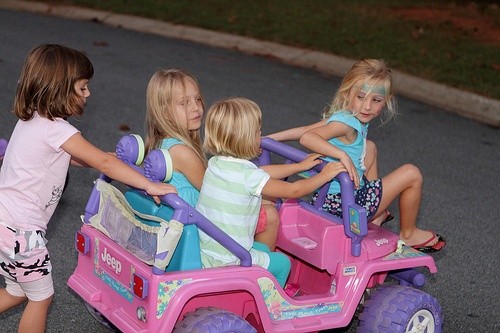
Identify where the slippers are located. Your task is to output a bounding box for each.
[411,231,446,253]
[380,210,394,227]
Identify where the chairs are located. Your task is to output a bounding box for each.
[124,190,205,271]
[287,159,400,261]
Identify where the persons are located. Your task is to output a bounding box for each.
[0,43,178,332]
[257,58,447,254]
[195,95,348,299]
[143,68,280,252]
